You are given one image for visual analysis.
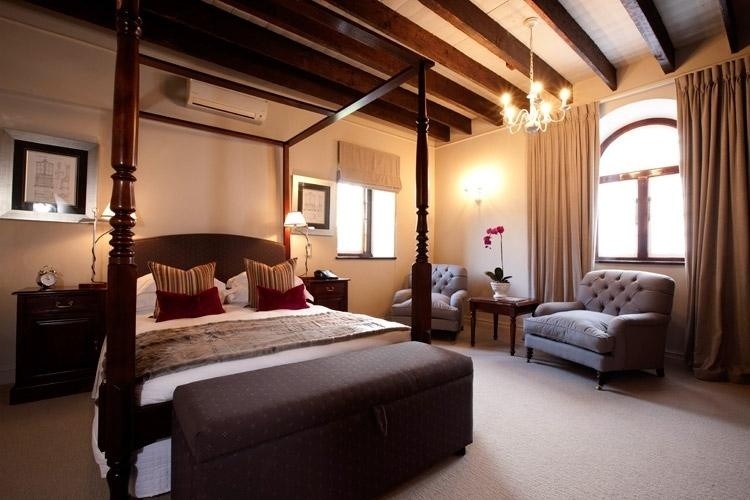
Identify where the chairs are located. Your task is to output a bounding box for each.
[521,267,676,391]
[389,263,469,342]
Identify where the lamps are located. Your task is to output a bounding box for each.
[495,14,573,138]
[283,211,315,280]
[78,204,138,288]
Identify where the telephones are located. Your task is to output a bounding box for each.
[314,269,338,279]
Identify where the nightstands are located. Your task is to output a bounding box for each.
[301,277,350,312]
[8,284,106,405]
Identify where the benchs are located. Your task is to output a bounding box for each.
[171,336,477,500]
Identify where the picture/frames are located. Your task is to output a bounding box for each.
[0,125,102,225]
[288,173,337,239]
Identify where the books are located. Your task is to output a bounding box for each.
[499,296,526,303]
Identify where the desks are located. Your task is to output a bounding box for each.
[466,295,542,358]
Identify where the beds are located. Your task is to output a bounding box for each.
[89,3,437,500]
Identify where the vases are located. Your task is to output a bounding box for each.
[489,282,511,299]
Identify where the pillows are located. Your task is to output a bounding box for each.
[256,284,311,311]
[154,287,227,324]
[241,257,300,310]
[227,267,317,307]
[136,270,228,315]
[145,260,218,318]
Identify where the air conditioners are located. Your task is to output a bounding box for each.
[181,72,272,126]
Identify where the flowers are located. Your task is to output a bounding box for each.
[482,224,514,282]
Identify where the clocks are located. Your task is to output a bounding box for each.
[34,264,61,290]
